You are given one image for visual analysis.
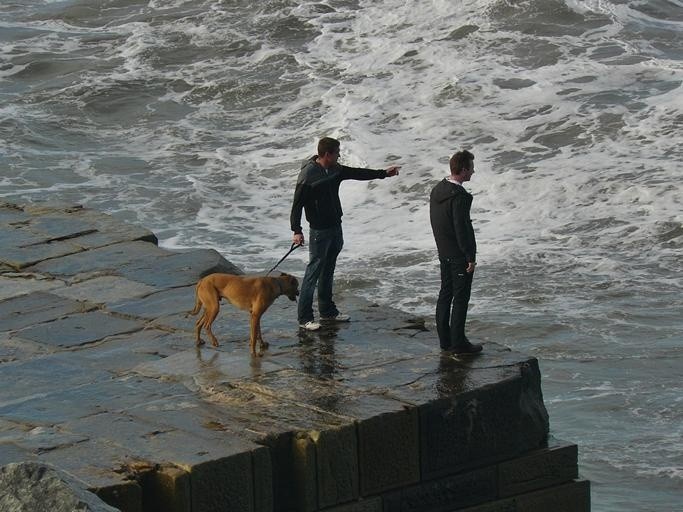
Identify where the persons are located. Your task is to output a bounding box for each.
[290,138,402,332]
[429,149,484,355]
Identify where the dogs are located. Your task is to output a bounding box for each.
[183,271,301,359]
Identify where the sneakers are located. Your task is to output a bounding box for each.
[320,310,351,322]
[299,320,322,331]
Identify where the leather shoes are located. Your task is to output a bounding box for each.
[439,344,451,350]
[451,340,483,354]
